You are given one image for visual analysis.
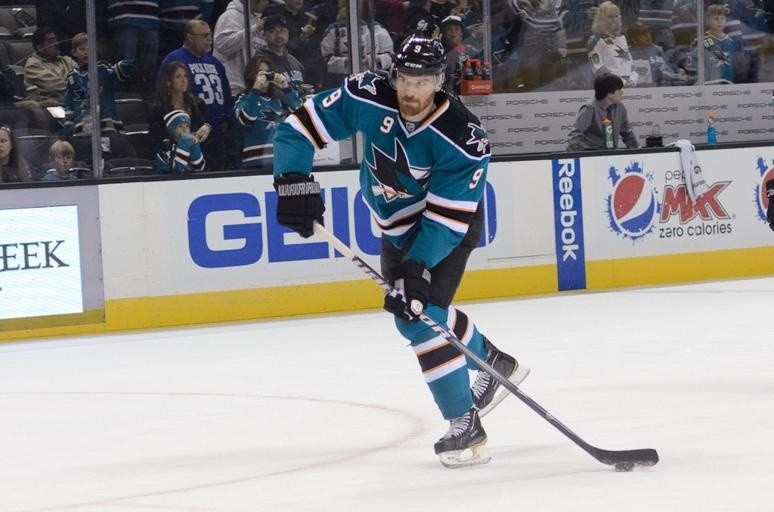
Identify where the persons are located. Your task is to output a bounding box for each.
[273,25,533,467]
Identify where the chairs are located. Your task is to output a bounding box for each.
[0,0,773,187]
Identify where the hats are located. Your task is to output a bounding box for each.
[263,12,289,31]
[161,108,193,135]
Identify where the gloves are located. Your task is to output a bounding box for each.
[378,256,433,322]
[271,170,327,240]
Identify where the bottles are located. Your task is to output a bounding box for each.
[707,117,717,146]
[602,117,616,149]
[462,59,491,82]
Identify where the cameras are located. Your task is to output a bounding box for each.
[262,72,275,81]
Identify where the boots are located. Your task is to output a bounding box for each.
[430,407,488,456]
[460,334,520,414]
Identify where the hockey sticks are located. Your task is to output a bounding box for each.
[313,223,658,468]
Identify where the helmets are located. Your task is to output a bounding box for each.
[389,30,449,77]
